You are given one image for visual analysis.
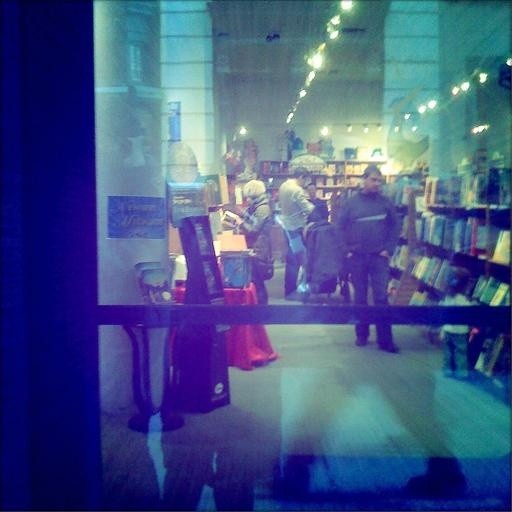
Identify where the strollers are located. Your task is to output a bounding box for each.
[303,220,352,303]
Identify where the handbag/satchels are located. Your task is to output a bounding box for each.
[288,232,305,253]
[255,258,274,280]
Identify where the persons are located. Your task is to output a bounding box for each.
[278,167,315,301]
[435,272,478,381]
[335,165,399,353]
[233,179,273,304]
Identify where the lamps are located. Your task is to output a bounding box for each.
[340,123,384,135]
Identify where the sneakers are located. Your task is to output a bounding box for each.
[378,342,399,353]
[355,336,367,346]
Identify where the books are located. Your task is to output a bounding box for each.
[228,136,385,222]
[225,211,239,219]
[385,172,511,376]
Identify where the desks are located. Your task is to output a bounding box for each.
[169,278,279,371]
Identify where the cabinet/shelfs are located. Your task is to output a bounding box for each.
[413,160,512,402]
[262,163,397,263]
[332,187,415,312]
